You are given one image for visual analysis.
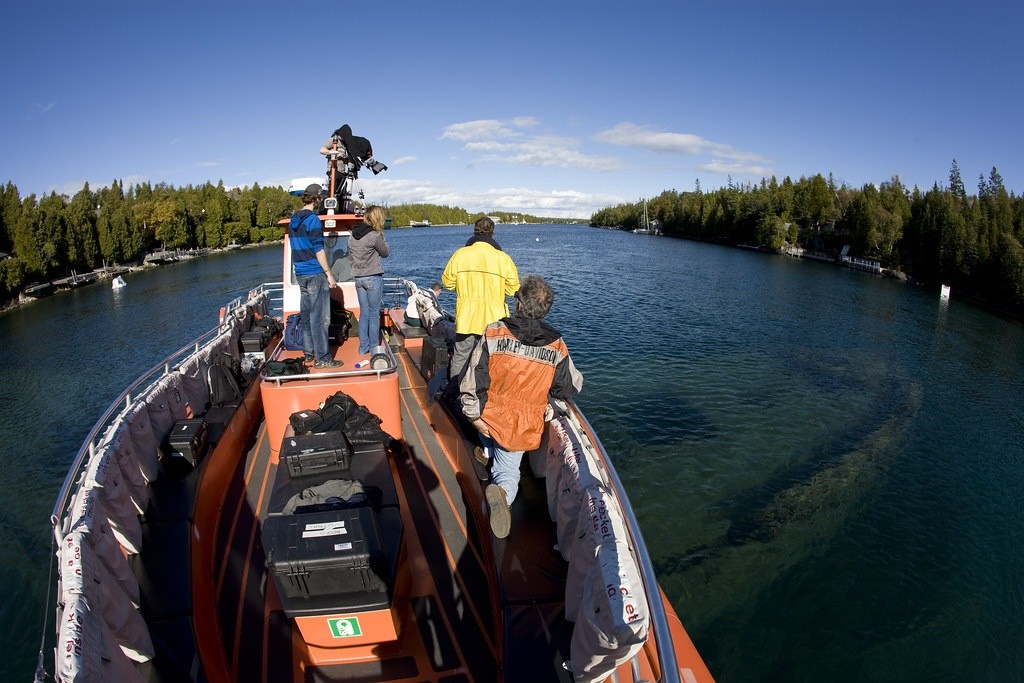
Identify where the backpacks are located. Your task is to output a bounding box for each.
[207,353,241,408]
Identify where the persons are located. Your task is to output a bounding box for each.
[444,217,521,378]
[458,276,584,538]
[347,205,390,354]
[404,283,442,326]
[289,184,344,369]
[318,130,346,214]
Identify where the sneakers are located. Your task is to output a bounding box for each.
[473,446,489,466]
[485,483,512,540]
[305,354,313,362]
[314,358,343,369]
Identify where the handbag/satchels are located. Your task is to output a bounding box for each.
[266,358,303,382]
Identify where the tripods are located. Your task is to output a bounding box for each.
[334,159,367,214]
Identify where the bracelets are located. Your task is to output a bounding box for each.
[325,269,330,272]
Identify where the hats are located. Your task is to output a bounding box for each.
[305,184,329,198]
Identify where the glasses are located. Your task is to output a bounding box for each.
[514,291,520,300]
